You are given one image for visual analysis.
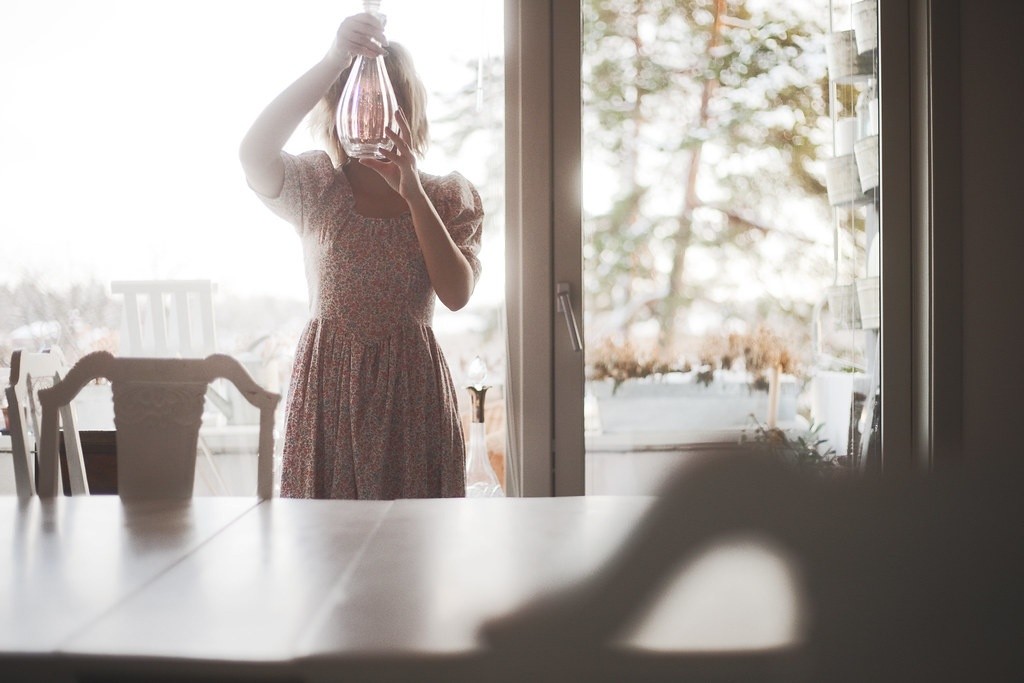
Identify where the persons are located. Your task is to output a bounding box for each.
[240,14,483,501]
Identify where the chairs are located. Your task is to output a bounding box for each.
[1,346,281,498]
[472,441,1024,683]
[111,276,237,428]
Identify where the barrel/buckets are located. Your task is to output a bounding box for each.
[825,153,868,207]
[855,276,880,329]
[853,157,881,194]
[828,286,862,331]
[825,0,877,81]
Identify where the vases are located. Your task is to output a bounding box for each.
[336,0,400,161]
[465,384,506,499]
[817,0,886,333]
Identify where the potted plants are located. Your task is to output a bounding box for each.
[587,318,801,435]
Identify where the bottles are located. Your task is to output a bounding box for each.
[464,385,505,498]
[336,0,400,159]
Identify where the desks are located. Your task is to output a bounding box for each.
[0,493,810,683]
